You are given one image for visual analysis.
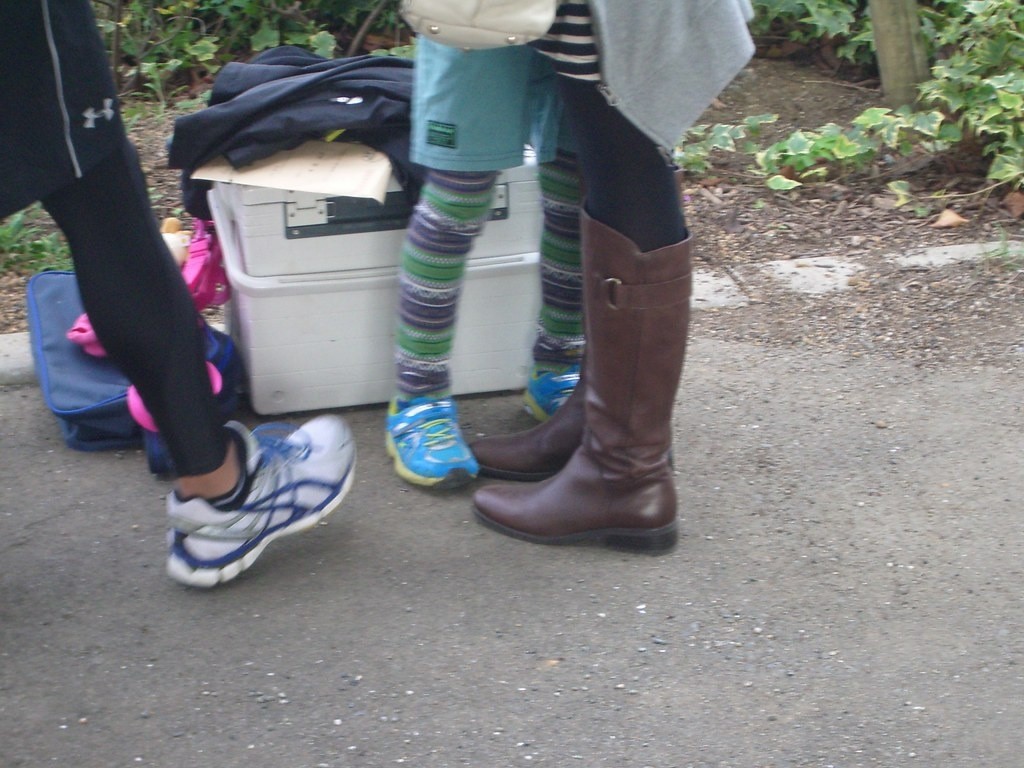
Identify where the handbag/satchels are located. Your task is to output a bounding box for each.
[27,257,239,450]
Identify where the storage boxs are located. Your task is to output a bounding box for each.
[207,188,541,416]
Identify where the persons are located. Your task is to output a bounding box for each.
[467,1,757,556]
[387,32,590,489]
[0,0,360,587]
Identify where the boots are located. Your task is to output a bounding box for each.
[471,197,696,552]
[467,361,590,478]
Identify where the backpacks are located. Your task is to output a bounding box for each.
[396,1,556,51]
[64,216,230,366]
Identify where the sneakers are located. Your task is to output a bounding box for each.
[378,393,481,493]
[159,417,357,590]
[522,359,588,423]
